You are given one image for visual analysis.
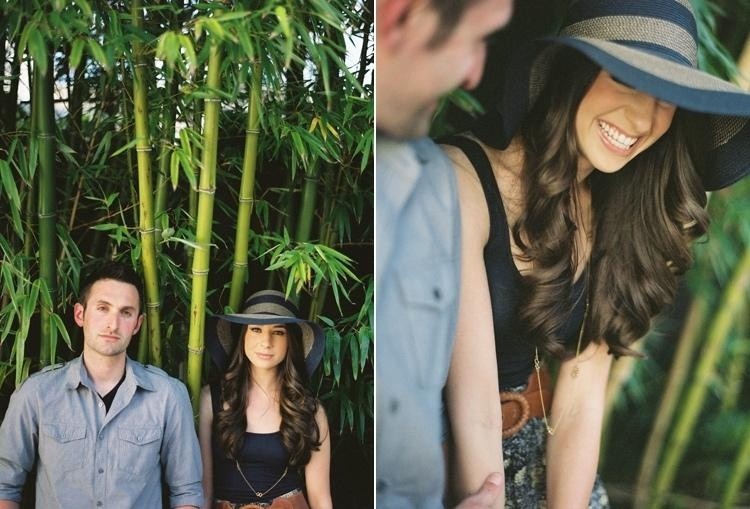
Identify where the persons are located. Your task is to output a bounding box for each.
[374,0,516,509]
[197,289,335,509]
[434,0,750,509]
[0,257,205,509]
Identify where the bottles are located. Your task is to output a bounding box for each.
[528,2,749,191]
[204,290,326,378]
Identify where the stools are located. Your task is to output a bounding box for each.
[443,368,552,461]
[215,491,309,507]
